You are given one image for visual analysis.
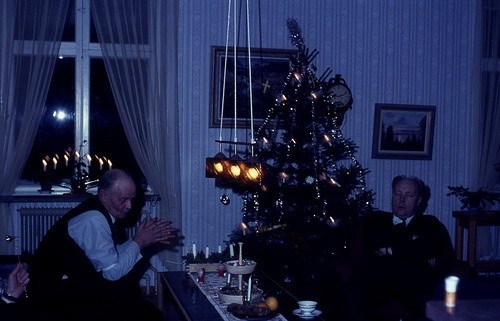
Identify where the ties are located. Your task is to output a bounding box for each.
[396,220,407,238]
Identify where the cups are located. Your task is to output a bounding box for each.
[298,300,316,315]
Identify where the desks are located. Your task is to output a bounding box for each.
[157,269,324,321]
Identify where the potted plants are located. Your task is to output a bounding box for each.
[446,186,500,212]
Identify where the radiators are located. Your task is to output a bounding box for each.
[17,206,141,254]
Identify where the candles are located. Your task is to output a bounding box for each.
[227,272,230,283]
[205,245,209,258]
[193,242,196,258]
[218,243,221,253]
[247,276,252,302]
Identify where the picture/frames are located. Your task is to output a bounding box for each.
[370,102,437,161]
[210,45,297,130]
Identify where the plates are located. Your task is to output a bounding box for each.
[293,309,322,318]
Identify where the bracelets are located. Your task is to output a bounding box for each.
[3,290,21,304]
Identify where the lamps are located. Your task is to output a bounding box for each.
[205,0,266,189]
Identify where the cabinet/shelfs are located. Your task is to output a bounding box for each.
[452,210,500,273]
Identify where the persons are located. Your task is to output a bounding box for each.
[28,168,177,321]
[0,262,30,321]
[337,175,454,321]
